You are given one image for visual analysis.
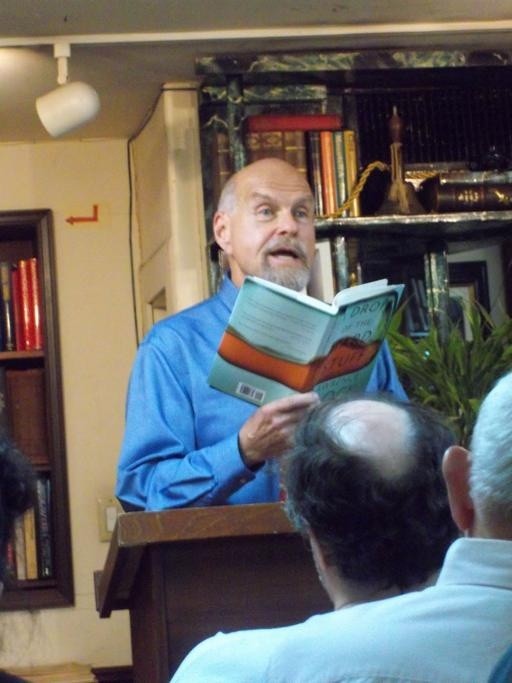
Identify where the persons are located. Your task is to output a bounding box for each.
[115,157,410,512]
[166,370,512,683]
[283,389,459,610]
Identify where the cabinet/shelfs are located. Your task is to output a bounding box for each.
[127,83,510,364]
[0,209,75,613]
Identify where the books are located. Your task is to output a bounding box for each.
[204,275,404,409]
[200,115,511,220]
[0,257,55,580]
[206,232,511,339]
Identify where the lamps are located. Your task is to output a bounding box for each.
[34,43,102,137]
